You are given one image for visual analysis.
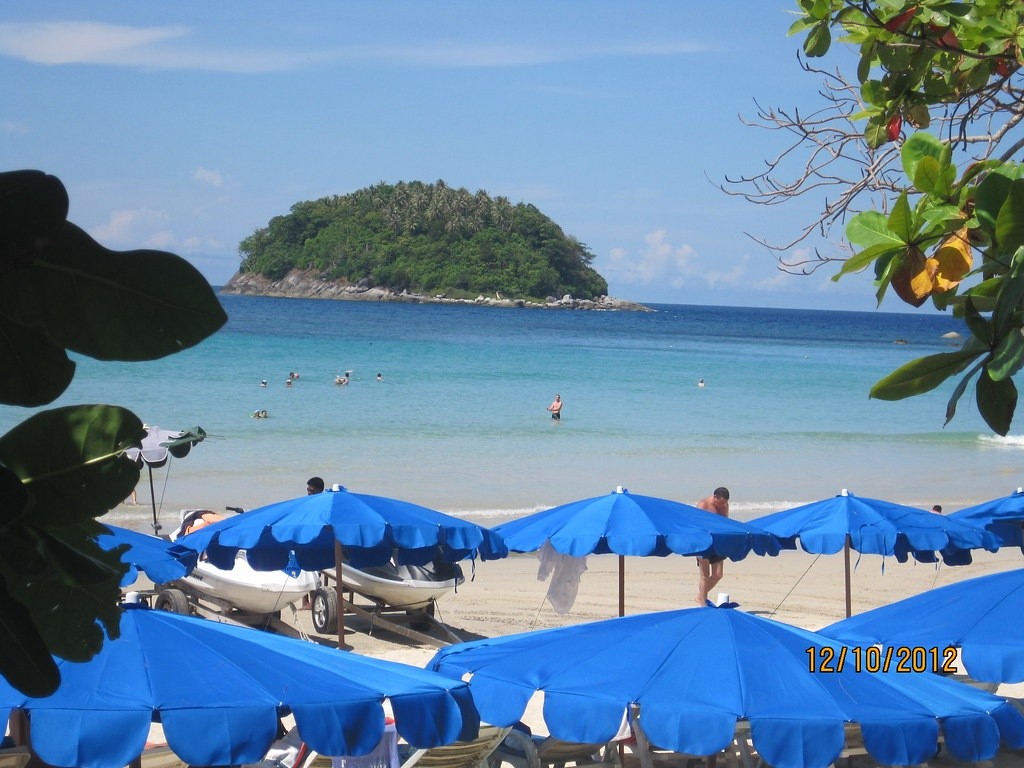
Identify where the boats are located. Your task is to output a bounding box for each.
[311,551,469,652]
[152,510,321,645]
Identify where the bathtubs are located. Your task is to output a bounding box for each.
[317,550,471,610]
[169,522,330,626]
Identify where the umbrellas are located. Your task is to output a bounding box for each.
[0,483,1024,768]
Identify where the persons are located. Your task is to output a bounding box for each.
[335,372,350,385]
[307,477,324,494]
[260,379,267,387]
[294,372,299,378]
[261,409,268,418]
[286,380,292,387]
[375,373,382,381]
[251,409,260,418]
[546,393,562,420]
[289,372,294,378]
[697,378,705,388]
